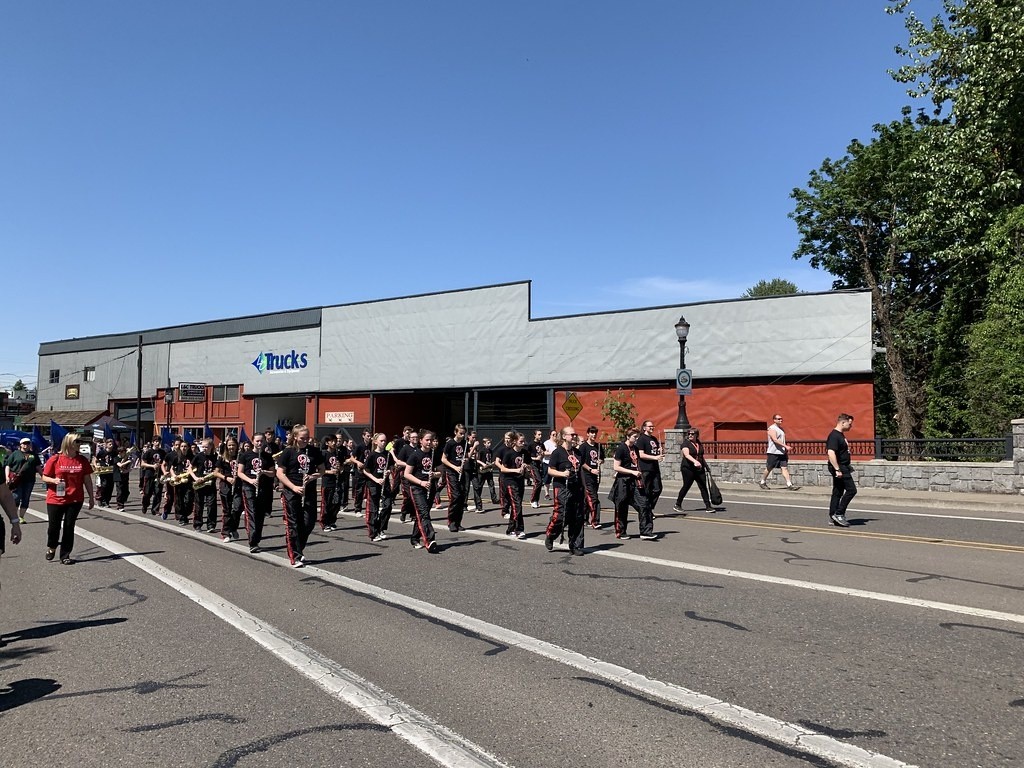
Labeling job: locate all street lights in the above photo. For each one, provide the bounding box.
[674,315,692,430]
[16,396,22,430]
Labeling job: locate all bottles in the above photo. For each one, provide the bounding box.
[57,479,65,496]
[96,475,101,485]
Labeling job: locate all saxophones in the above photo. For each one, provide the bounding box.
[170,471,189,487]
[193,472,215,490]
[272,450,283,458]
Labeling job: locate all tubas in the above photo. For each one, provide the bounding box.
[119,444,139,470]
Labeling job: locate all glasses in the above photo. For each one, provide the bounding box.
[687,432,695,435]
[564,433,578,437]
[775,418,782,421]
[74,439,84,445]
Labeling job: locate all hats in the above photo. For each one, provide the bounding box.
[19,437,31,444]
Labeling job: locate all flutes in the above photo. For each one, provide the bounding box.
[228,429,675,508]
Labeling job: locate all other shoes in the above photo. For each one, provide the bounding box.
[503,513,511,520]
[546,539,553,550]
[788,485,800,490]
[674,505,684,513]
[339,505,483,522]
[292,556,305,567]
[428,540,437,554]
[508,531,526,540]
[98,501,262,553]
[640,534,658,539]
[706,508,715,513]
[372,531,386,542]
[573,548,584,556]
[758,481,770,491]
[45,547,56,561]
[324,524,337,532]
[59,558,71,564]
[620,534,630,540]
[584,521,601,530]
[414,542,423,550]
[450,523,465,531]
[530,501,540,508]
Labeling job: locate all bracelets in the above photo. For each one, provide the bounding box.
[835,468,840,472]
[10,517,20,524]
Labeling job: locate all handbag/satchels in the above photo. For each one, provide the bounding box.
[706,471,723,505]
[8,471,20,483]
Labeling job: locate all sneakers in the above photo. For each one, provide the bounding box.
[828,510,851,527]
[19,516,27,524]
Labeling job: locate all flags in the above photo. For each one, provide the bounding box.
[50,418,69,451]
[104,421,113,439]
[239,427,250,443]
[184,429,195,444]
[275,423,287,443]
[32,422,50,451]
[130,431,136,446]
[161,427,178,448]
[205,421,213,439]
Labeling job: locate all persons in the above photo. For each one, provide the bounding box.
[758,414,801,491]
[92,420,665,569]
[42,431,94,565]
[826,413,857,527]
[0,461,22,648]
[42,450,51,490]
[5,438,43,524]
[672,428,716,513]
